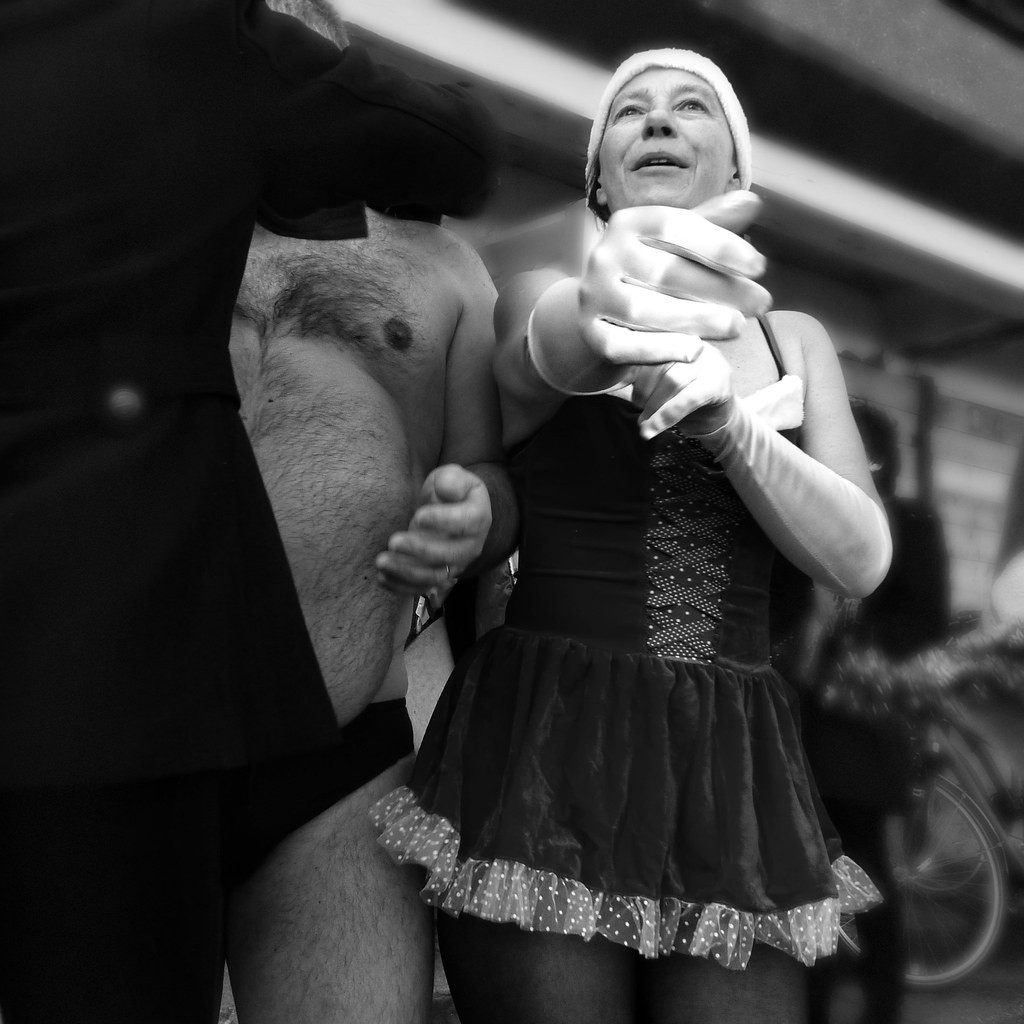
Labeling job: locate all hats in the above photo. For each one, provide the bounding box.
[582,42,753,225]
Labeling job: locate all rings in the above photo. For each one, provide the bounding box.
[443,563,451,581]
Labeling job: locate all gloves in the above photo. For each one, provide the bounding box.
[526,190,774,394]
[617,343,893,600]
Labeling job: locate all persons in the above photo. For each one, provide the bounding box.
[0,1,499,1024]
[222,0,514,1024]
[370,46,884,1024]
[772,399,951,1024]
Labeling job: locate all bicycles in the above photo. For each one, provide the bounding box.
[823,618,1024,990]
